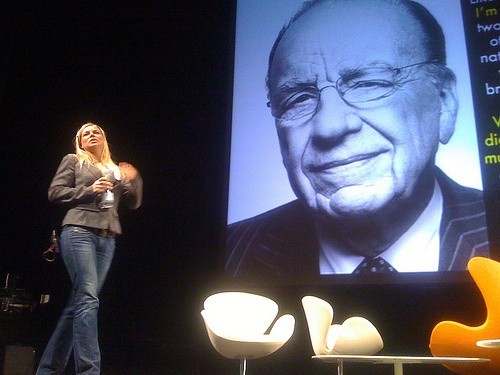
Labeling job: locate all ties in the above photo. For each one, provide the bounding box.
[350,257,399,274]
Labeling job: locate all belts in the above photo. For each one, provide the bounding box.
[73,225,118,239]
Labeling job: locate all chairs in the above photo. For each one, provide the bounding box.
[201,292,295,375]
[301,295,384,375]
[429,257,500,375]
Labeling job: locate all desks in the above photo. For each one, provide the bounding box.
[312,354,491,375]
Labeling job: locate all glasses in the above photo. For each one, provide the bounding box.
[266,58,440,120]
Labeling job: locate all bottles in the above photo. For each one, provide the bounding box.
[102,171,114,208]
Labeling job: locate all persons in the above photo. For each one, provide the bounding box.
[225,0,489,276]
[36,122,143,375]
